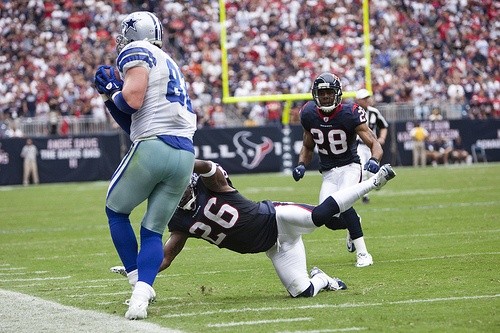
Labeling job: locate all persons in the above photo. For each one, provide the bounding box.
[93,9,198,320]
[20,138,40,185]
[428,137,473,166]
[352,88,389,201]
[288,73,383,269]
[0,0,500,136]
[111,158,397,300]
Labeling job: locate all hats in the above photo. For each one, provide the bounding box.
[356,88,371,101]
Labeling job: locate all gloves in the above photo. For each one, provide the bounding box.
[93,65,112,92]
[363,159,380,174]
[293,164,305,181]
[108,265,127,278]
[96,66,124,99]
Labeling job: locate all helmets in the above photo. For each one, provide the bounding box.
[120,11,163,47]
[312,73,343,113]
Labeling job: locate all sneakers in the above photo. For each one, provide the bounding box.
[125,280,156,319]
[375,164,394,191]
[310,266,348,291]
[355,252,373,267]
[346,216,362,252]
